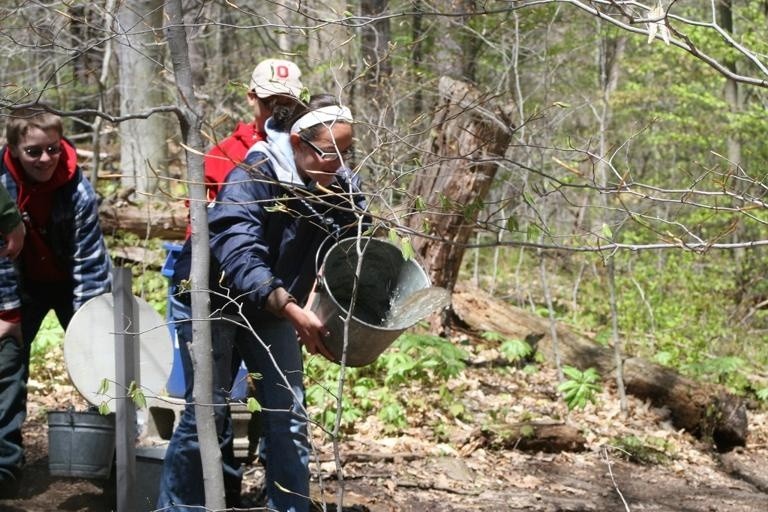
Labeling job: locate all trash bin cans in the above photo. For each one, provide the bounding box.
[161,242,251,398]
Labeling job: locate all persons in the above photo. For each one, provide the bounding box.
[0,105,117,501]
[185,59,310,247]
[1,181,28,264]
[155,90,375,511]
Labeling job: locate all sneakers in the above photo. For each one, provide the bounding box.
[1,473,18,499]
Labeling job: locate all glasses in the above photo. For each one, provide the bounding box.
[26,143,60,157]
[300,135,353,162]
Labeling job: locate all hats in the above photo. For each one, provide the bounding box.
[250,58,304,99]
